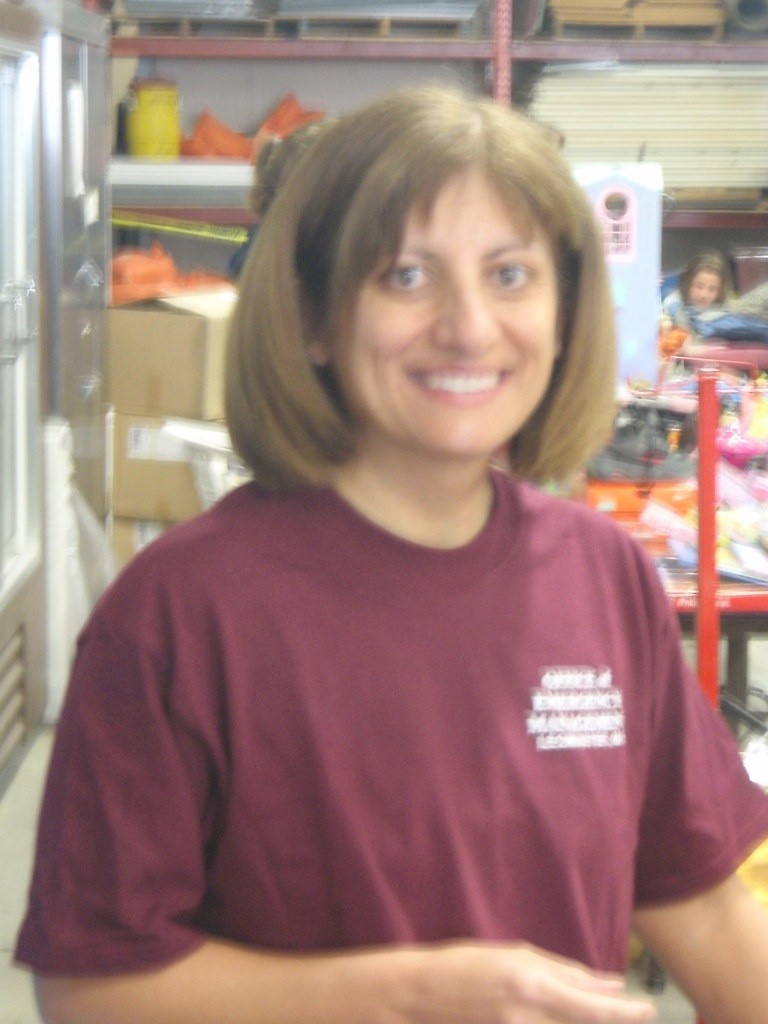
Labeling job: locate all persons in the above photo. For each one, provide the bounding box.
[10,87,768,1024]
[228,118,334,269]
[660,254,726,322]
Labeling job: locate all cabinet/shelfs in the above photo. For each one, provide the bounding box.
[111,40,768,227]
[0,0,114,796]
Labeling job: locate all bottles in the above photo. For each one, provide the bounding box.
[665,413,682,451]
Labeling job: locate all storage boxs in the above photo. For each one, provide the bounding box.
[110,287,251,573]
